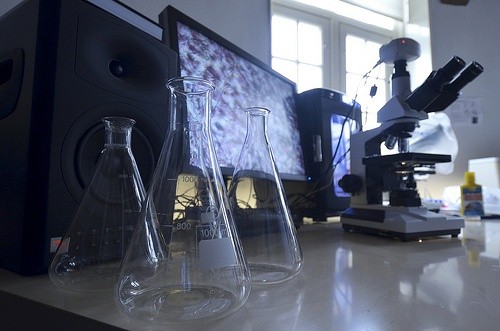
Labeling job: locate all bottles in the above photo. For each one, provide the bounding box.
[111,73,252,324]
[204,106,302,285]
[48,117,173,301]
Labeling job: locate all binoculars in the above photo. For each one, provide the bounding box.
[340,37,484,243]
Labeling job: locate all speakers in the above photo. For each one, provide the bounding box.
[0,0,179,273]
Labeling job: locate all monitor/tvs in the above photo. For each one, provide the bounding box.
[158,4,314,193]
